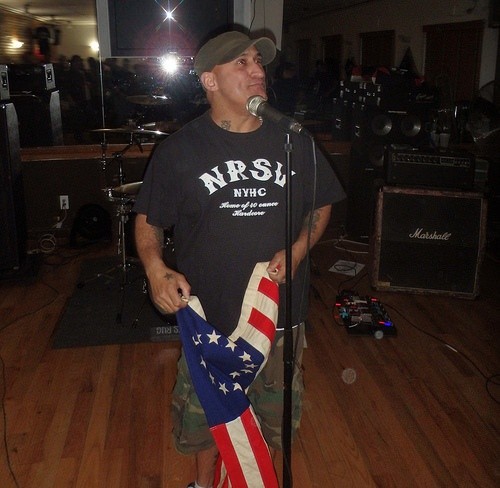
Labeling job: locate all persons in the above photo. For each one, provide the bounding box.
[131,31,350,488]
[8,53,209,145]
[265,54,299,113]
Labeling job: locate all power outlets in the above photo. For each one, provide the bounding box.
[59,195,69,209]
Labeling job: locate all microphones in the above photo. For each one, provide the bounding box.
[245,94,313,138]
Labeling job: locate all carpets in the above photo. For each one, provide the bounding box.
[50,254,181,348]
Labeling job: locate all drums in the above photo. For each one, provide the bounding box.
[102,189,141,205]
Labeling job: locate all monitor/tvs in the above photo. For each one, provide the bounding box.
[96,0,251,58]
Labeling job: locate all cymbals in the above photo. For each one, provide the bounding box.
[143,121,184,131]
[127,94,173,106]
[91,127,170,137]
[116,181,145,194]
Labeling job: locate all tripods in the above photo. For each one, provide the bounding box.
[77,132,145,324]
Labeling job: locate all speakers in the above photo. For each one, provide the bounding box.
[13,91,66,147]
[345,108,433,241]
[0,102,29,280]
[369,181,489,302]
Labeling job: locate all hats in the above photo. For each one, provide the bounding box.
[194,32,277,77]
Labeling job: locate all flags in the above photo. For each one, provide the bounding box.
[175,261,281,488]
